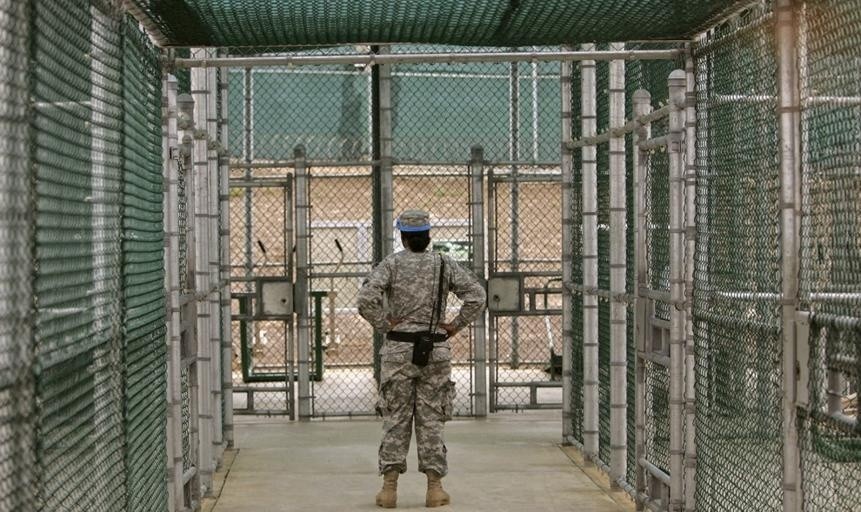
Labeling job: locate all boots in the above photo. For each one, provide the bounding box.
[376,470,399,508]
[426,470,450,507]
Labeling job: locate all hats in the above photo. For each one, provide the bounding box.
[396,210,431,232]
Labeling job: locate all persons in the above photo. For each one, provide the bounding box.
[356,209,488,509]
